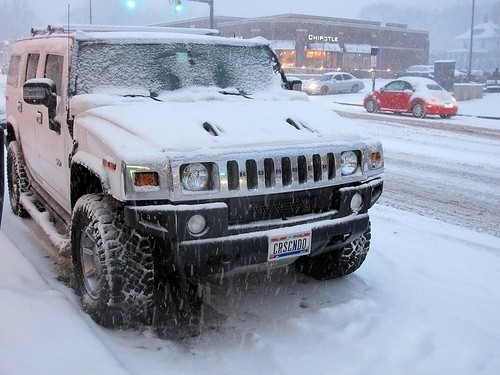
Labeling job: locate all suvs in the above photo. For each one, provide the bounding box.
[0,25,384,329]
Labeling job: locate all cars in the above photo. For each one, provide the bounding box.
[363,76,458,119]
[301,73,365,95]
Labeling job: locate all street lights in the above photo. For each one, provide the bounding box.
[175,0,214,34]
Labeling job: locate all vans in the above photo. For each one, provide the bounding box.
[395,65,433,78]
